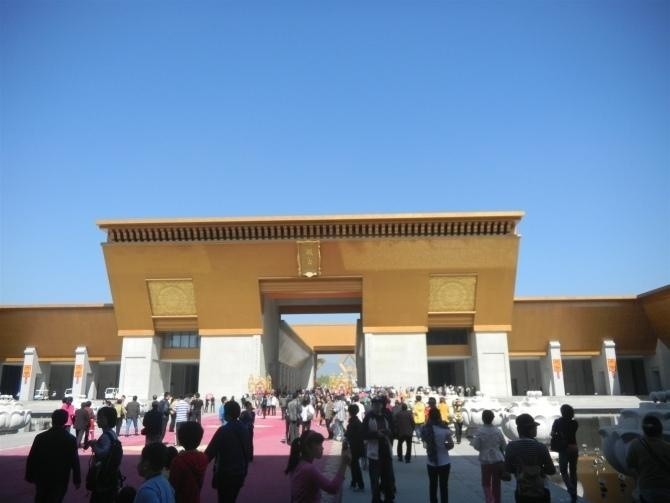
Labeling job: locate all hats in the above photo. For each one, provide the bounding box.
[516,414,540,426]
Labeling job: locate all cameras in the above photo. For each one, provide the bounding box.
[342,440,351,450]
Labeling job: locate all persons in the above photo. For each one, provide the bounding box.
[624,414,670,503]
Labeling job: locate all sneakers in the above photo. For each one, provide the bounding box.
[349,484,365,492]
[398,456,411,463]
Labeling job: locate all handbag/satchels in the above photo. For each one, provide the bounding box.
[551,438,560,451]
[104,440,122,471]
[86,471,116,492]
[497,462,510,481]
[342,438,352,464]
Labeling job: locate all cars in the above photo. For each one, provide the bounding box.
[104,387,117,400]
[115,387,119,399]
[64,387,72,400]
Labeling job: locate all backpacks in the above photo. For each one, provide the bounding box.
[512,440,545,497]
